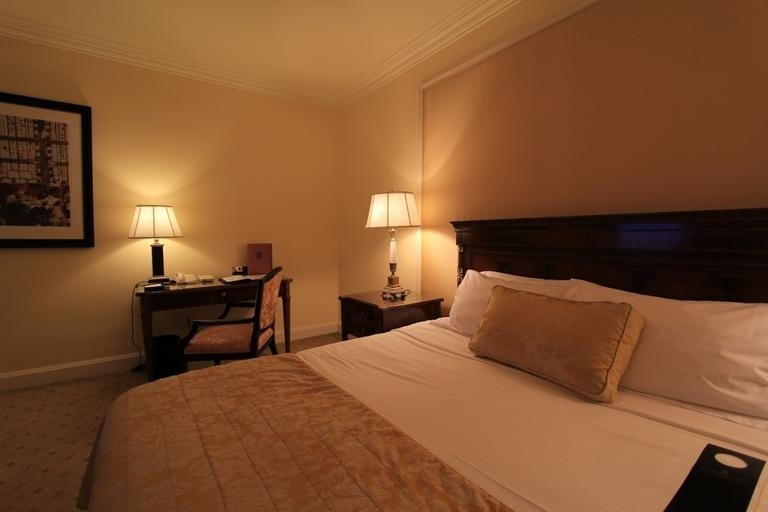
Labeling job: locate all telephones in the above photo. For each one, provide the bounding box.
[175,273,196,283]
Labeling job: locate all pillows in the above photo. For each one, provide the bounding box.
[448,269,571,337]
[562,283,767,418]
[469,285,644,405]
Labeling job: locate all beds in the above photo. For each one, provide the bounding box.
[77,208,768,512]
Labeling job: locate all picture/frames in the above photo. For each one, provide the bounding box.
[0,92,95,248]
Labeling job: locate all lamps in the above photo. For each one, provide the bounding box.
[365,191,422,302]
[128,205,183,277]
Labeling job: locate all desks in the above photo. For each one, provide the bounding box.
[134,273,293,382]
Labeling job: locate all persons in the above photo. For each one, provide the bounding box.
[2,195,28,225]
[23,200,53,225]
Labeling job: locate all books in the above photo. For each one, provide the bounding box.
[218,271,267,284]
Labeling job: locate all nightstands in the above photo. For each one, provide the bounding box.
[338,289,443,342]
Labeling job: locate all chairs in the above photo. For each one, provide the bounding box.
[176,266,282,374]
